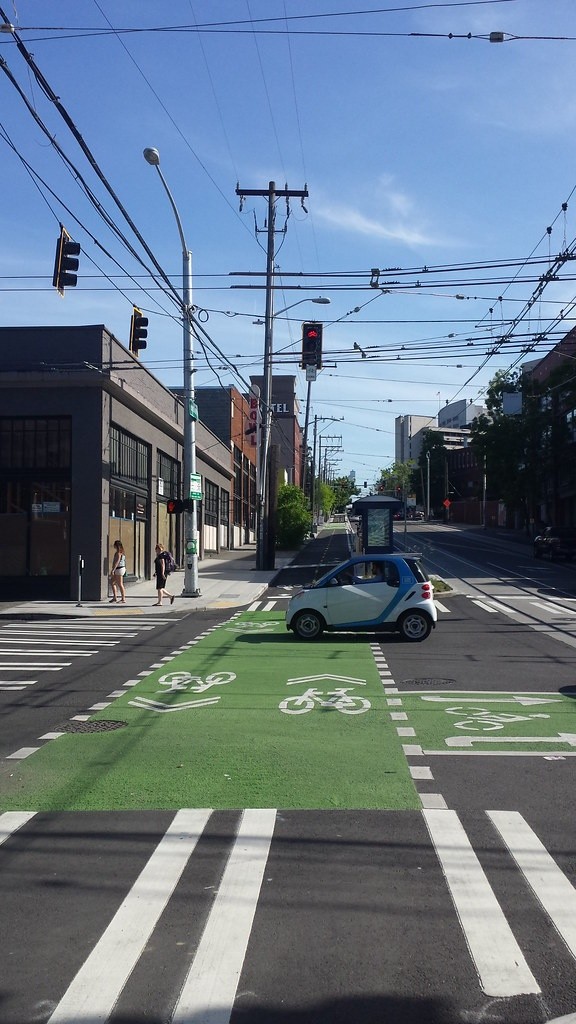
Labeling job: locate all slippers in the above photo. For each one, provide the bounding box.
[171,596,175,604]
[153,604,162,606]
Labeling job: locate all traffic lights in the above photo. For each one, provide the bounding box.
[379,485,385,492]
[300,322,322,370]
[396,486,401,492]
[55,228,81,300]
[129,307,149,357]
[166,499,185,516]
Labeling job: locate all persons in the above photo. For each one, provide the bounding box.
[109,540,127,604]
[345,562,384,583]
[151,543,175,606]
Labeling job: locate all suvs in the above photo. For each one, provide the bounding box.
[533,525,576,563]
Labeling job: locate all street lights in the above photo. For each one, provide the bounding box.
[144,146,203,598]
[255,297,332,571]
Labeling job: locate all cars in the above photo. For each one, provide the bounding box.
[393,507,444,520]
[285,551,438,644]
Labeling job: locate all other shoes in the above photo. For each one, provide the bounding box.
[116,600,126,603]
[108,599,117,603]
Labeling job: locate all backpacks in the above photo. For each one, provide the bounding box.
[158,550,175,573]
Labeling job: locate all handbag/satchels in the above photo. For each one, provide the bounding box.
[123,571,127,577]
